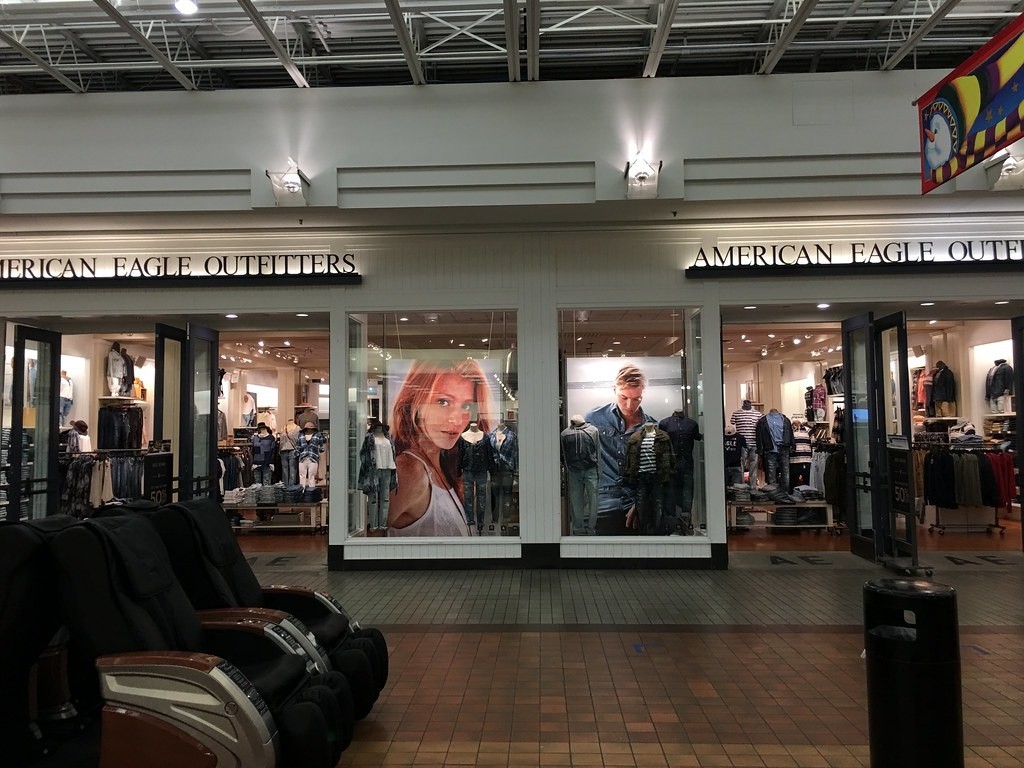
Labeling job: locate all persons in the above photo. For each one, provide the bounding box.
[489,422,518,524]
[106,347,135,396]
[730,400,764,490]
[560,415,602,533]
[804,383,827,421]
[383,358,491,537]
[757,408,795,490]
[59,370,73,427]
[933,362,957,417]
[359,418,399,537]
[298,407,321,431]
[625,423,676,535]
[250,422,276,486]
[295,422,325,488]
[584,363,660,535]
[243,392,256,423]
[923,367,939,418]
[278,419,303,485]
[456,422,498,531]
[985,359,1015,414]
[658,409,704,535]
[724,424,749,487]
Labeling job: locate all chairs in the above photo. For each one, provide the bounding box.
[0,496,388,768]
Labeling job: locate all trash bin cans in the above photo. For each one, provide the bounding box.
[862,577,966,768]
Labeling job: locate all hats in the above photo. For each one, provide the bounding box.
[70,420,88,436]
[255,422,267,430]
[299,422,318,433]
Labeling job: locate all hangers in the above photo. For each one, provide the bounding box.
[100,402,143,412]
[59,449,147,464]
[810,447,851,463]
[912,435,1011,459]
[218,448,252,462]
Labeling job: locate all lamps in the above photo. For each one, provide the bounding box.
[249,344,300,364]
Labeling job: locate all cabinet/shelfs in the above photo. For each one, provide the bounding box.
[728,500,834,536]
[983,412,1021,507]
[223,498,329,537]
